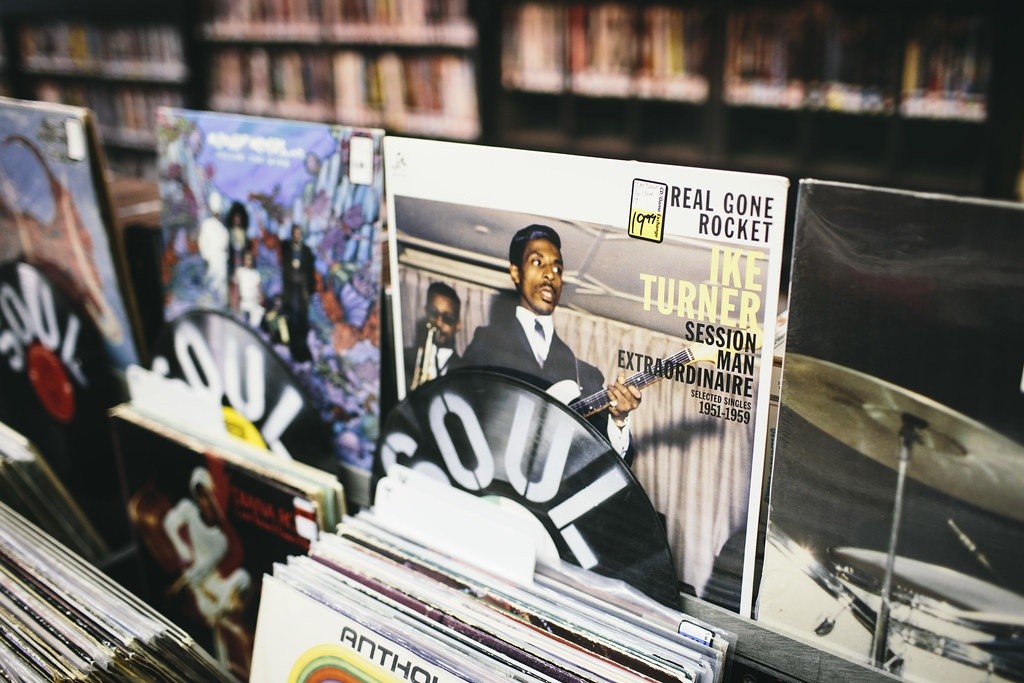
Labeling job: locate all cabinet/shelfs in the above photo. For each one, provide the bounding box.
[24,0,1024,184]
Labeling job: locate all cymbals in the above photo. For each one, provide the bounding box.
[780,352,1024,523]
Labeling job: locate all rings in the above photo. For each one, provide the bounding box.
[610,400,617,406]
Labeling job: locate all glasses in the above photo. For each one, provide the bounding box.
[426,304,456,326]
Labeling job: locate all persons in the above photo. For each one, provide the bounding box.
[460,224,642,467]
[404,282,461,394]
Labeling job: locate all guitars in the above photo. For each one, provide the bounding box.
[544,325,764,419]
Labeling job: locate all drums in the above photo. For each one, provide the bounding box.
[828,545,1024,648]
[886,621,1024,682]
[756,520,903,672]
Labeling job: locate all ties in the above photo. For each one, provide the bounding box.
[534,319,546,341]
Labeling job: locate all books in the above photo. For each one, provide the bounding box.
[202,0,469,119]
[498,0,709,106]
[721,0,995,122]
[18,20,185,131]
